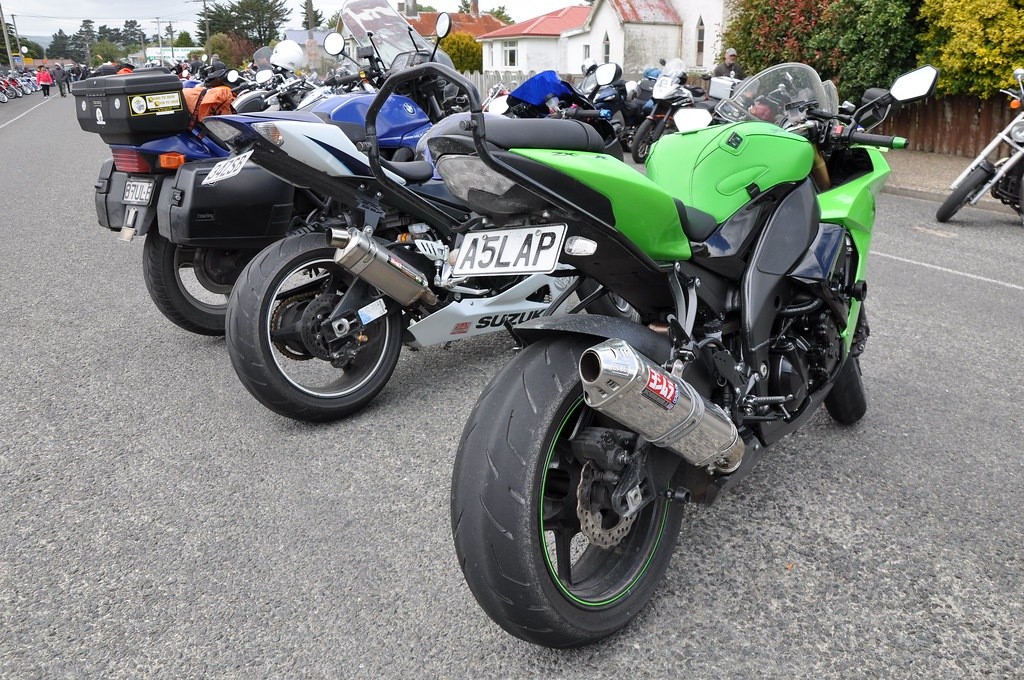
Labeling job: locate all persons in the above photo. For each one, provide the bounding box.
[65,60,129,83]
[52,63,69,96]
[169,51,210,82]
[36,65,53,98]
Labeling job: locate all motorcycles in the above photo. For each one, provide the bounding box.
[0,0,1024,650]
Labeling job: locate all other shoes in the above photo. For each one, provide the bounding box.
[45,96,49,98]
[69,91,71,93]
[62,94,66,97]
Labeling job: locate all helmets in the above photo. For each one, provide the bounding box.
[269,40,304,71]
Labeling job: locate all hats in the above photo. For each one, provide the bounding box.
[212,54,220,59]
[726,48,737,55]
